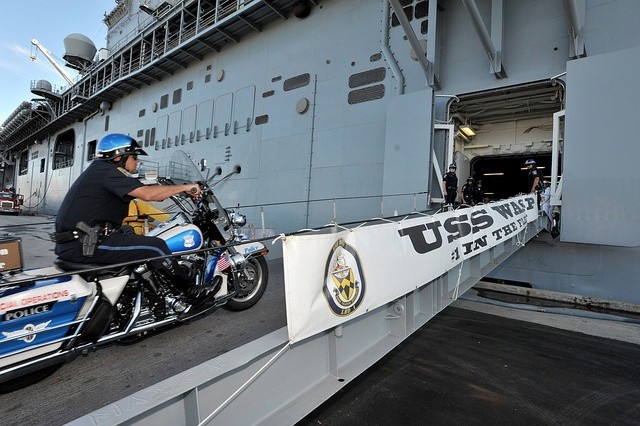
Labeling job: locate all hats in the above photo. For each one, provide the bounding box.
[450,162,454,167]
[525,157,535,164]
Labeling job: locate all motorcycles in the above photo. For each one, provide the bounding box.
[0,149,268,382]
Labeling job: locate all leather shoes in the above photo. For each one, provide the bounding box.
[187,275,223,309]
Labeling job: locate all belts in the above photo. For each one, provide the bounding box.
[50,230,86,242]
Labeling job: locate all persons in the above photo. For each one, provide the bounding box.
[523,158,542,192]
[542,180,550,196]
[473,179,486,202]
[49,132,224,308]
[461,176,475,206]
[442,163,458,209]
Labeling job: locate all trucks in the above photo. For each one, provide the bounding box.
[0,191,24,214]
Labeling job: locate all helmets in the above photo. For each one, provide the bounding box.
[94,133,148,161]
